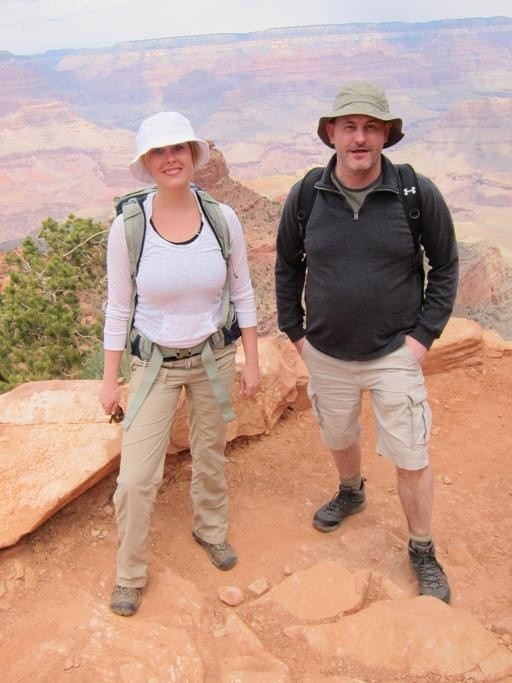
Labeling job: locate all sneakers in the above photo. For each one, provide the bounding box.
[195,534,237,570]
[408,543,452,604]
[111,587,139,616]
[315,481,368,533]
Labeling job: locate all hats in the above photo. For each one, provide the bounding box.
[129,111,208,183]
[316,81,404,149]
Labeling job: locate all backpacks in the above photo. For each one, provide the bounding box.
[113,184,239,362]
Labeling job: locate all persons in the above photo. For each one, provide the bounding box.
[96,108,262,616]
[274,79,458,605]
